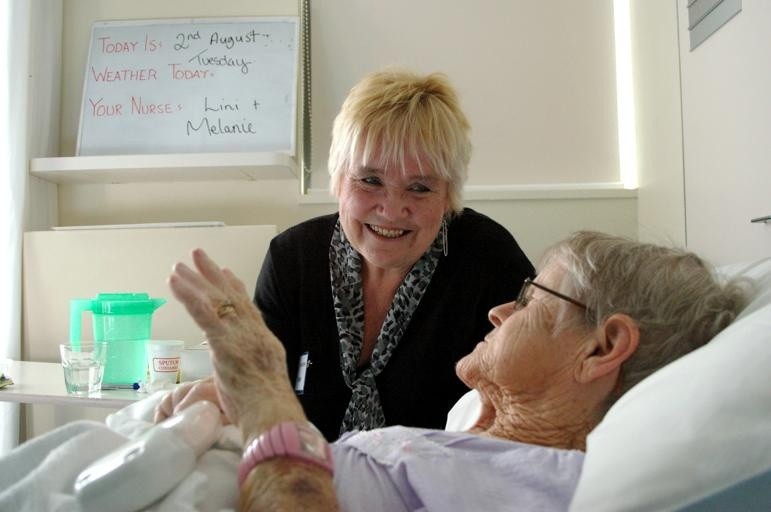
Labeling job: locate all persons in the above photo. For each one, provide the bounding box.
[253,65,537,444]
[153,230,763,512]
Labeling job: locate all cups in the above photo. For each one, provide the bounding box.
[67,290,168,389]
[59,341,108,397]
[145,338,183,388]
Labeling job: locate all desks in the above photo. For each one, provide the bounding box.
[0,358,150,409]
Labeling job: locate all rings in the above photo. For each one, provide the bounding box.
[217,304,235,317]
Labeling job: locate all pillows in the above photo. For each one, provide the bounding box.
[444,264,771,511]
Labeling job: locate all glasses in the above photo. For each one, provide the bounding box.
[515,278,590,313]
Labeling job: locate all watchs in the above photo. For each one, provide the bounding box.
[238,418,336,489]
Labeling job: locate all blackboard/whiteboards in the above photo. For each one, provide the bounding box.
[75,16,301,157]
